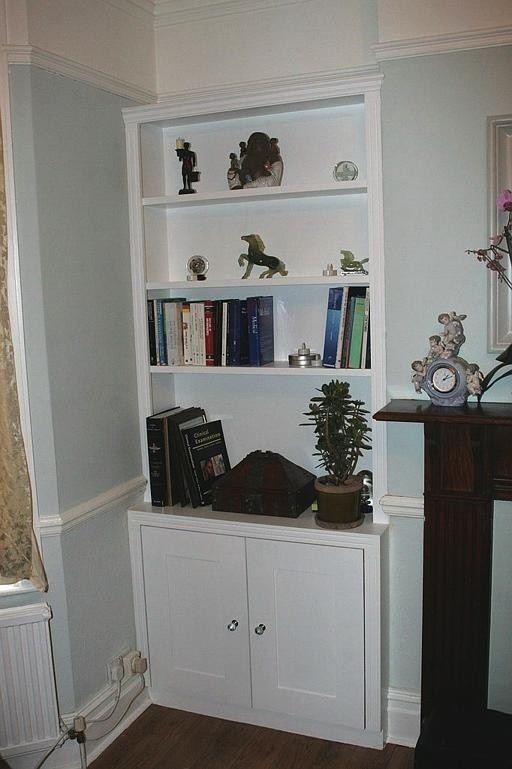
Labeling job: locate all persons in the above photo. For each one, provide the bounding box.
[270,137,280,155]
[438,313,461,351]
[229,152,239,170]
[411,360,428,394]
[178,142,196,190]
[425,335,443,358]
[466,363,484,396]
[239,141,247,157]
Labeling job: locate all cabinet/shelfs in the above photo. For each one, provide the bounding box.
[128,500,390,751]
[118,74,383,519]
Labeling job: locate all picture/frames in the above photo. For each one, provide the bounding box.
[487,113,512,354]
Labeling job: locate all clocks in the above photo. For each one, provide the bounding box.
[411,312,482,407]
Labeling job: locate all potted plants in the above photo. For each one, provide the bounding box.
[298,379,372,529]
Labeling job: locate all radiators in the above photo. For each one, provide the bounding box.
[0,603,65,759]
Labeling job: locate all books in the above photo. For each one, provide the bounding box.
[145,404,233,508]
[322,286,370,369]
[147,294,274,367]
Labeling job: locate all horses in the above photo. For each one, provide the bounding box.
[238,235,288,279]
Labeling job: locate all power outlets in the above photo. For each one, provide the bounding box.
[105,647,139,684]
